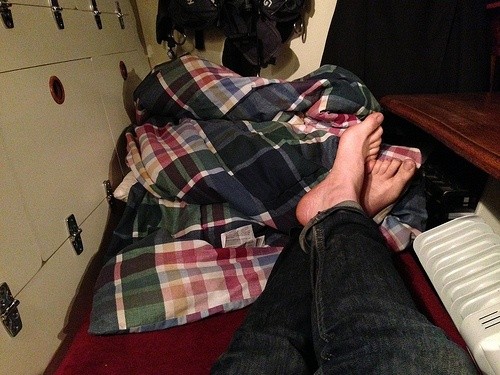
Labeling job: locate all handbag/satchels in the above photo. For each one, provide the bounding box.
[156,0,310,76]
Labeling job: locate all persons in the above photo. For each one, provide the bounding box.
[212,113,480,375]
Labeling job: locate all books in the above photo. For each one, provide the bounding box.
[406,145,494,217]
